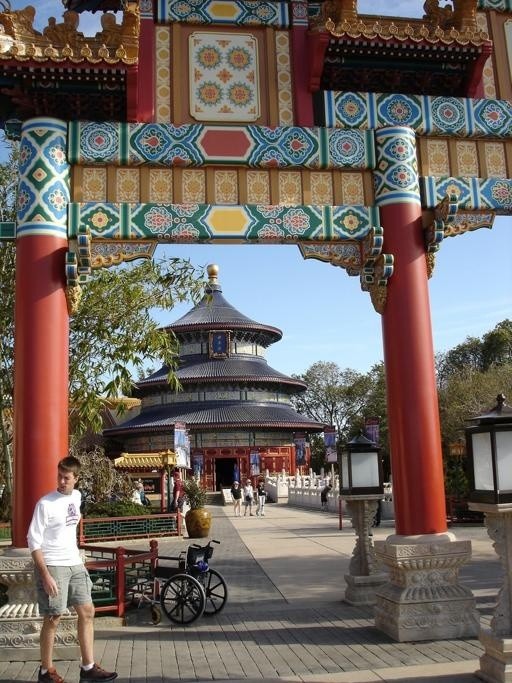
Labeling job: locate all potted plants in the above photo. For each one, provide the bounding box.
[178,477,211,537]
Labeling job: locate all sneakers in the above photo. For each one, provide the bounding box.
[37,665,66,683]
[79,663,118,683]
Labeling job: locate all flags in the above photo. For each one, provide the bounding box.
[364,415,379,447]
[193,452,204,475]
[294,438,307,465]
[174,420,192,470]
[249,450,261,476]
[323,425,337,463]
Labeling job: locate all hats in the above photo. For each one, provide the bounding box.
[232,478,265,485]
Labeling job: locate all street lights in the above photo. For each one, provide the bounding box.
[464,393,512,683]
[339,430,388,607]
[159,449,176,513]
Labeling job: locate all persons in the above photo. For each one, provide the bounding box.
[26,453,118,682]
[131,480,143,505]
[231,480,242,518]
[256,478,268,516]
[170,472,185,514]
[243,478,255,516]
[138,482,146,506]
[320,483,333,512]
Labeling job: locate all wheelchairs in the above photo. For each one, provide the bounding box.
[138,540,227,624]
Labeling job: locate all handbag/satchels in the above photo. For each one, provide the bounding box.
[245,495,251,501]
